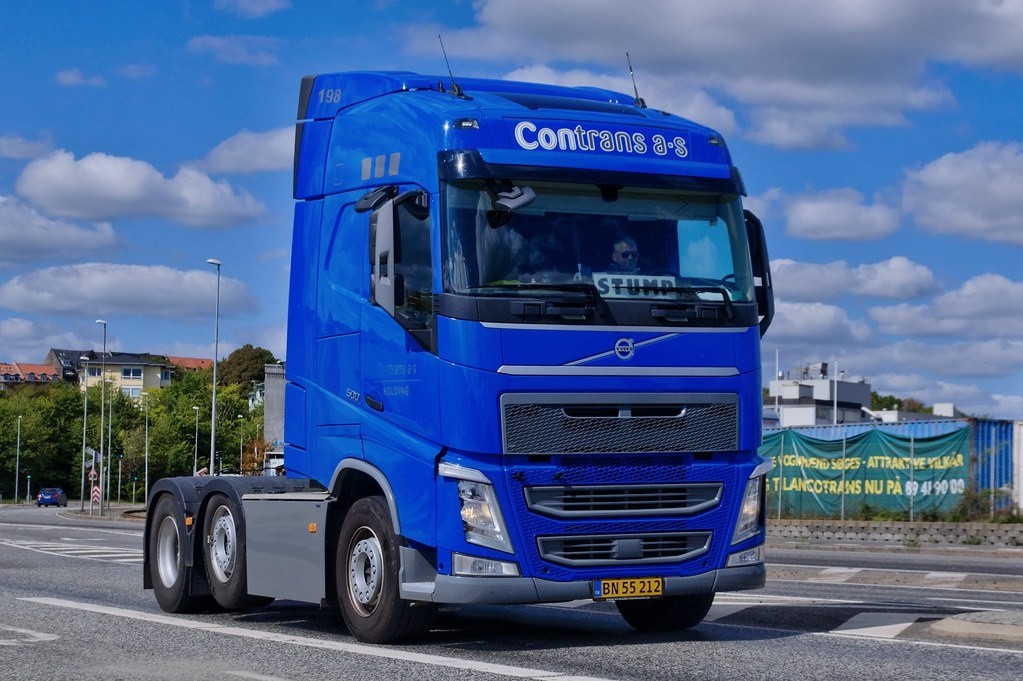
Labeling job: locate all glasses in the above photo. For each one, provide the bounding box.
[614,250,639,259]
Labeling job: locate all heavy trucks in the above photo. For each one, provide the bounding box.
[142,64,776,646]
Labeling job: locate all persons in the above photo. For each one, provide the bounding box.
[609,236,641,274]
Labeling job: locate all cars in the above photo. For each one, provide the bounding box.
[217,467,240,476]
[36,487,68,507]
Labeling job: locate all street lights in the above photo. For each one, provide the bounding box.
[192,406,199,476]
[96,318,107,516]
[141,391,149,506]
[104,379,113,512]
[206,257,222,476]
[237,414,244,475]
[80,355,89,512]
